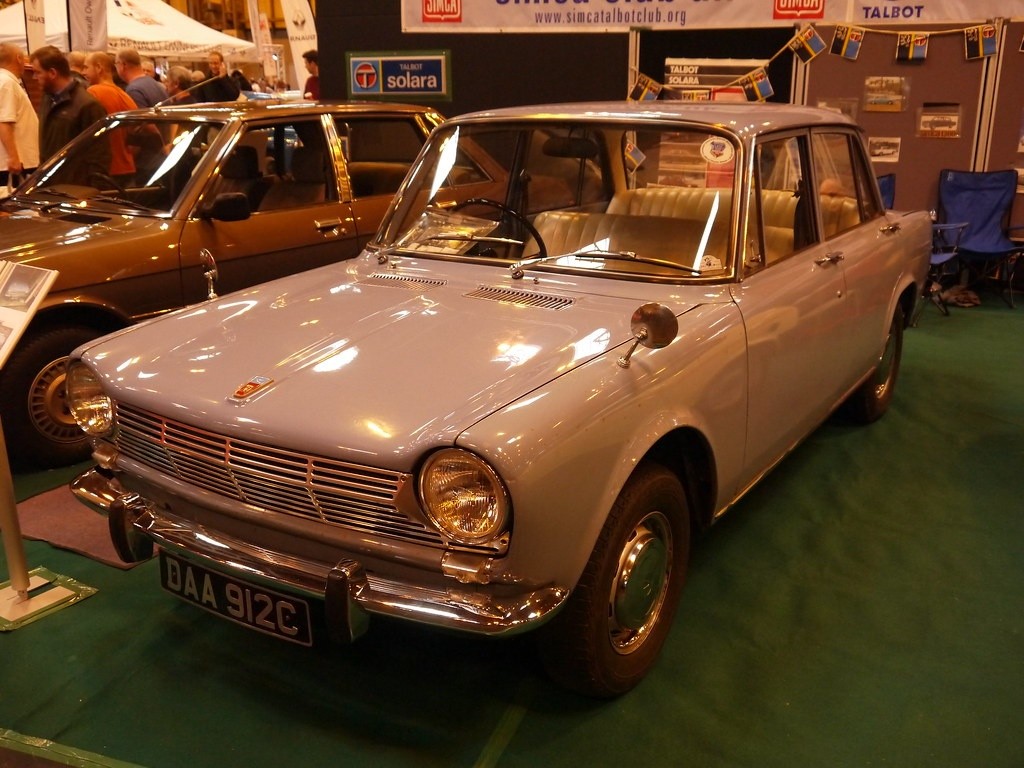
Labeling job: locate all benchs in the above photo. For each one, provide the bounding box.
[518,209,795,277]
[603,187,861,269]
[344,160,469,200]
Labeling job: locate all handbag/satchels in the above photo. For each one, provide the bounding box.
[0,173,24,199]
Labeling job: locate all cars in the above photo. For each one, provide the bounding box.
[0,102,454,469]
[64,100,935,703]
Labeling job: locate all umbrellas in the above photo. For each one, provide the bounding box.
[0,0,259,65]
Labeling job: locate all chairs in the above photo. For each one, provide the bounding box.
[255,145,336,211]
[199,144,265,212]
[875,169,1024,327]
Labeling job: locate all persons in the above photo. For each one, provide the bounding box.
[165,65,197,104]
[67,50,166,188]
[191,53,289,103]
[212,144,297,214]
[0,41,40,189]
[303,49,321,101]
[29,45,110,189]
[107,50,167,109]
